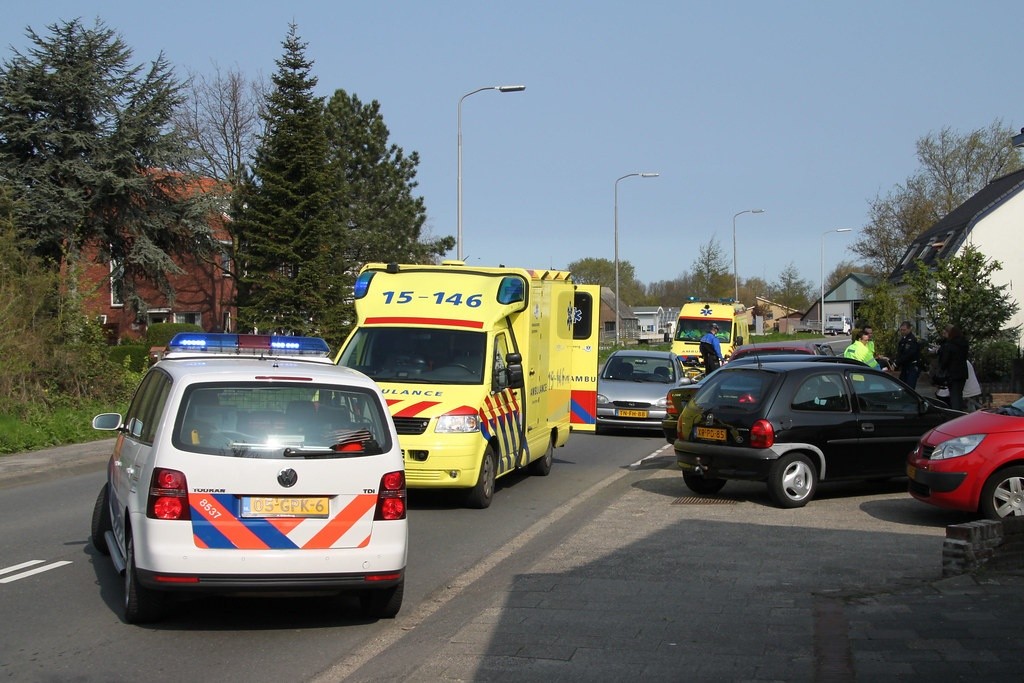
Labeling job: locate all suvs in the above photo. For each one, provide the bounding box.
[673,361,970,508]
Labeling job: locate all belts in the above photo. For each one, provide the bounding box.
[911,363,919,366]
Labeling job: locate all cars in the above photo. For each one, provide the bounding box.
[906,396,1024,519]
[595,349,691,433]
[661,354,949,445]
[728,342,827,363]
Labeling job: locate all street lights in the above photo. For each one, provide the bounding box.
[821,228,852,336]
[734,208,765,300]
[457,85,526,263]
[615,173,660,344]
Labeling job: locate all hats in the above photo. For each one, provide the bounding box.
[711,323,720,331]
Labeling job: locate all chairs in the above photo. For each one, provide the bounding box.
[819,382,846,409]
[615,362,634,380]
[285,401,351,443]
[653,367,670,380]
[446,335,481,374]
[384,334,427,370]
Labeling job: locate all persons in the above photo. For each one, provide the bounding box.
[891,321,919,392]
[843,324,889,393]
[699,322,726,376]
[938,323,982,413]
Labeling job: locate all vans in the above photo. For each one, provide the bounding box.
[91,333,407,625]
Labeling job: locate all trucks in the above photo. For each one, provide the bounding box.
[333,261,601,508]
[791,313,852,336]
[671,297,750,379]
